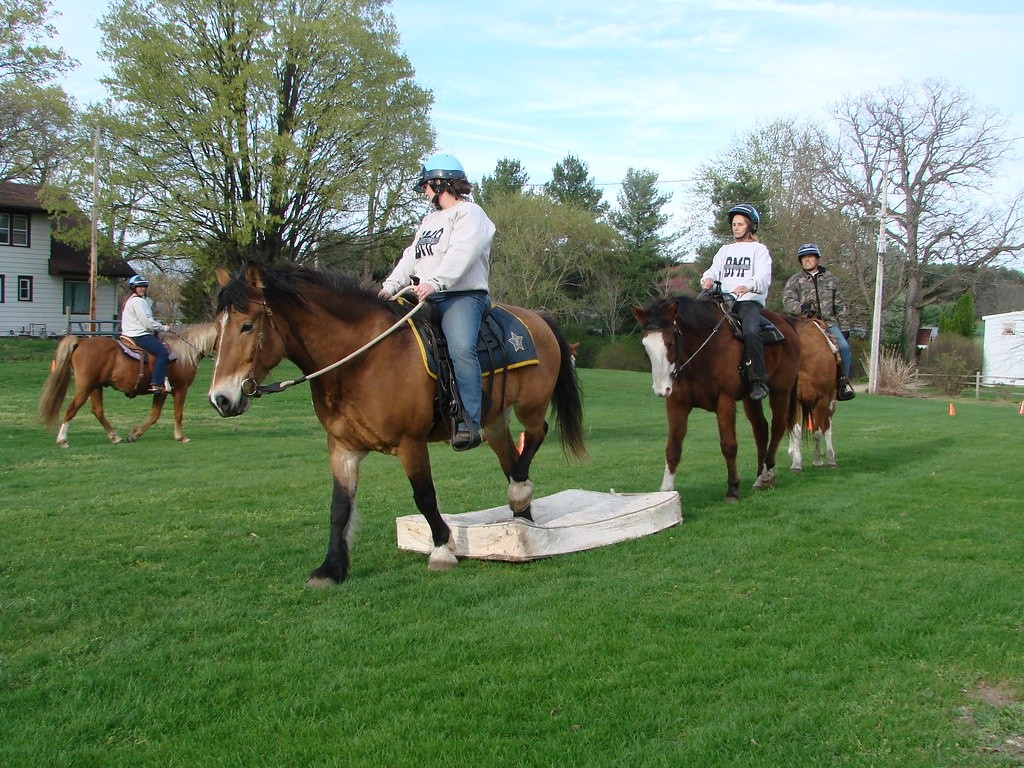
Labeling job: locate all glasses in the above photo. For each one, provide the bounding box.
[422,183,428,190]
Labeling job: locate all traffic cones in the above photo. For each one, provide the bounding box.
[1019,400,1024,414]
[949,403,956,416]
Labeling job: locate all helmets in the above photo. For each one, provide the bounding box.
[728,204,759,223]
[129,275,149,288]
[412,154,465,193]
[797,244,821,262]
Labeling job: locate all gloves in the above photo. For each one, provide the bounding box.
[842,331,850,340]
[801,303,811,311]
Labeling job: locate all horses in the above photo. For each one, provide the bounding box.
[35,322,218,449]
[628,296,839,504]
[208,259,590,587]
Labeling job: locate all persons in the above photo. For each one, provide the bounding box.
[701,204,773,400]
[378,153,497,445]
[782,244,854,402]
[121,274,171,392]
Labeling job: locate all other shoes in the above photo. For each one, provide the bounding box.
[841,388,855,397]
[454,431,487,448]
[151,384,174,389]
[751,383,770,400]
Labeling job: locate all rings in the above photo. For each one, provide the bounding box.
[421,290,426,296]
